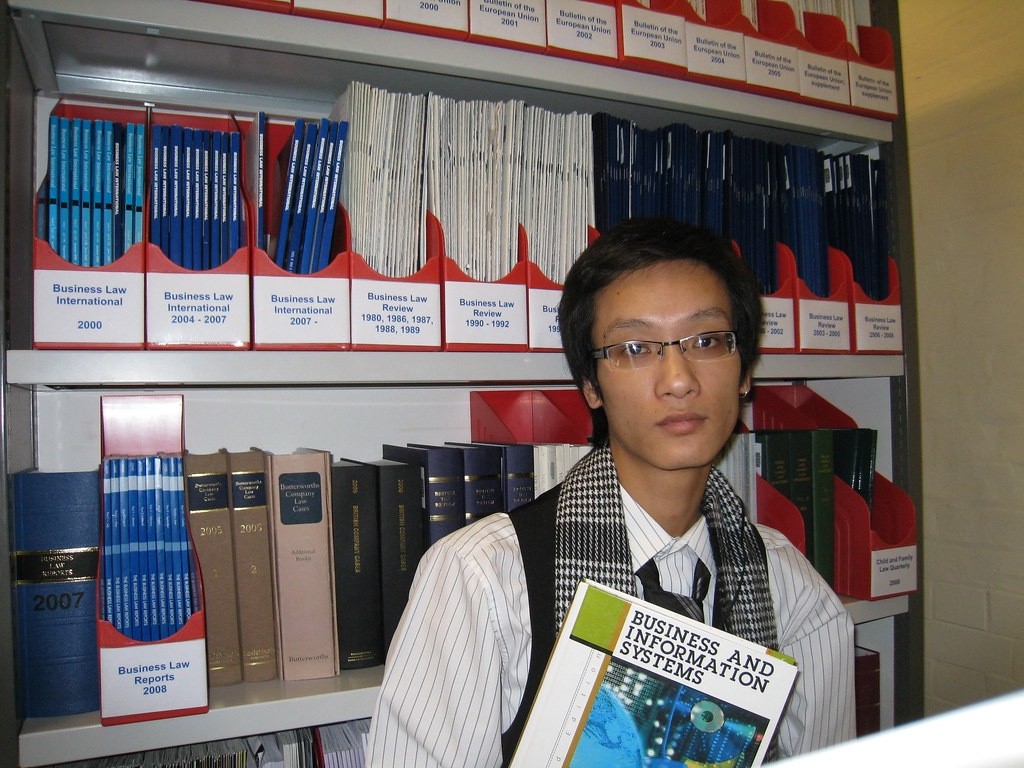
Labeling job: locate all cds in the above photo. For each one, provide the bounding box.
[664,685,757,763]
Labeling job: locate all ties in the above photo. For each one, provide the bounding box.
[635,556,712,624]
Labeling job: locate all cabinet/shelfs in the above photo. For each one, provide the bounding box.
[0,0,920,768]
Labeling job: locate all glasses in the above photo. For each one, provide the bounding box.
[590,329,737,370]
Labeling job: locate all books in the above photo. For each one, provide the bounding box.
[0,0,891,768]
[510,578,799,768]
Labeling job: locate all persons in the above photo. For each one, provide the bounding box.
[368,214,856,768]
